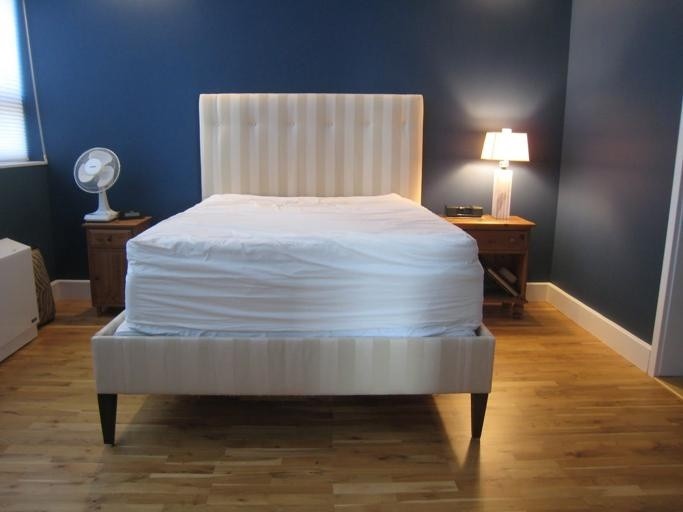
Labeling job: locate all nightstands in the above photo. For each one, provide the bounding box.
[440,215,536,319]
[82,216,153,317]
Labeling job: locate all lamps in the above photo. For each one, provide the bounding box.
[480,127,530,221]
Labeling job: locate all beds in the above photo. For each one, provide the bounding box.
[90,93,496,447]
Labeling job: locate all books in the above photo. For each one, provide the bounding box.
[486,269,520,298]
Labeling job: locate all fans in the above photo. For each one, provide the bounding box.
[73,147,121,223]
